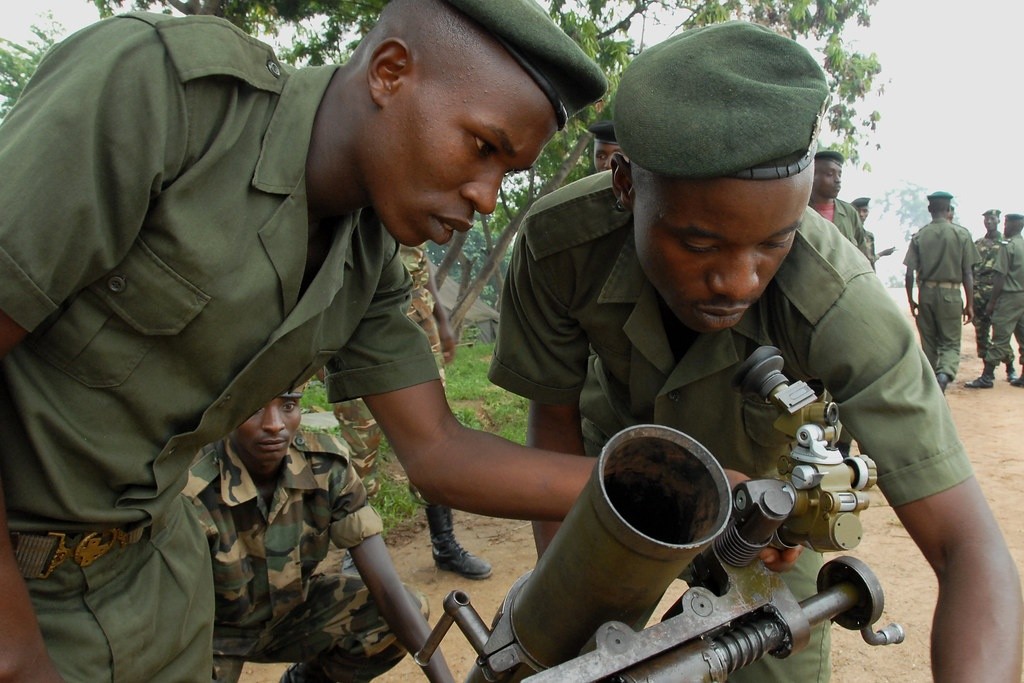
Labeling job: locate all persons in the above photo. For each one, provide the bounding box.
[587,121,629,172]
[972,209,1019,382]
[850,197,897,264]
[317,241,494,579]
[964,214,1024,388]
[903,191,983,395]
[180,380,457,683]
[807,152,876,273]
[0,0,804,683]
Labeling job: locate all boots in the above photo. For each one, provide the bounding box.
[965,363,994,388]
[425,503,493,580]
[1006,362,1015,381]
[937,372,950,392]
[1011,365,1024,387]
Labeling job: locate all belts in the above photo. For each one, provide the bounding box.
[925,282,960,289]
[11,522,144,579]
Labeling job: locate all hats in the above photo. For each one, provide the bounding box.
[815,151,844,168]
[928,191,952,204]
[982,209,1001,216]
[852,197,870,208]
[589,120,618,145]
[612,20,831,180]
[445,0,608,133]
[1004,214,1024,220]
